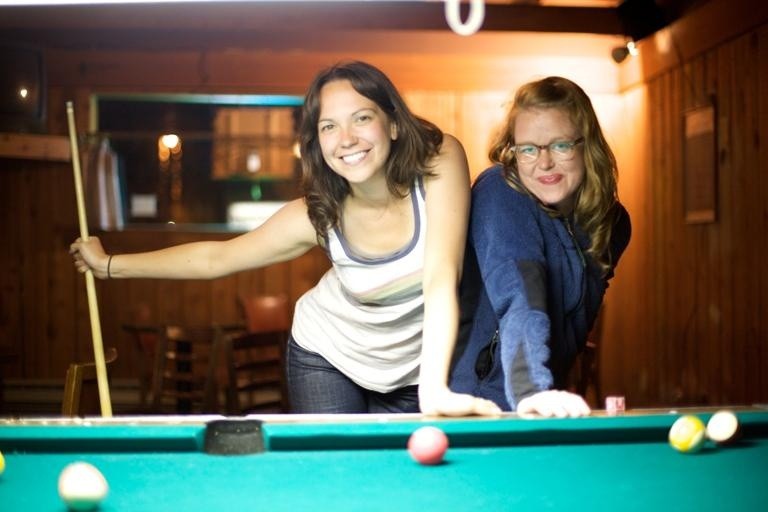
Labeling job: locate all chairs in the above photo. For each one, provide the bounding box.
[148,291,291,415]
[61,346,120,412]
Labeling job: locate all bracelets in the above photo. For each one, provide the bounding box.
[108,254,113,281]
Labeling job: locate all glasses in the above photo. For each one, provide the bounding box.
[511,137,583,164]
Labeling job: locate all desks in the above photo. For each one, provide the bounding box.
[0,402,767,512]
[125,322,246,413]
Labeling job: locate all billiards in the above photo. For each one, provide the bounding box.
[708,412,741,443]
[669,416,705,453]
[59,461,107,510]
[407,427,448,464]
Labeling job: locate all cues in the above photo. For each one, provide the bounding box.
[67,101,114,416]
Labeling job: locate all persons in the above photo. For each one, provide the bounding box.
[68,60,502,417]
[448,77,631,419]
